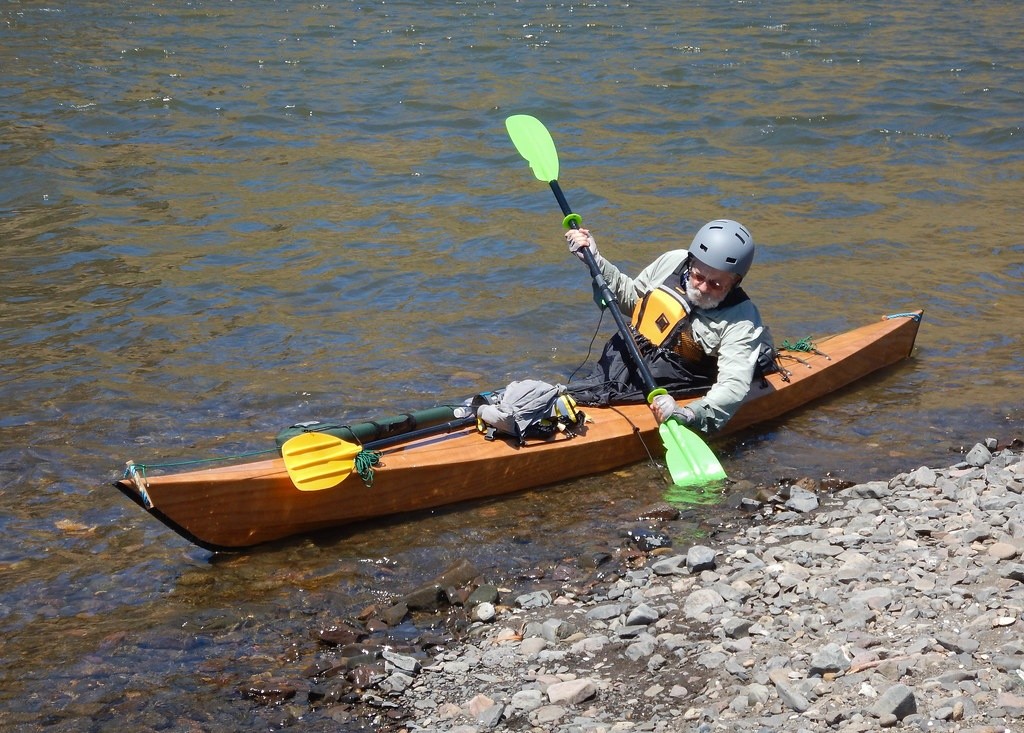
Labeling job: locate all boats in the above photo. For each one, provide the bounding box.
[110,307,928,555]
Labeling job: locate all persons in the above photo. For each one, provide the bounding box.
[564,219,764,437]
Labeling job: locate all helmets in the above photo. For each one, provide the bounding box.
[688,219,755,289]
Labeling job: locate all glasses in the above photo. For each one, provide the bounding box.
[689,270,723,292]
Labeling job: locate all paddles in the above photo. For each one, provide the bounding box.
[504,112,728,490]
[280,416,478,493]
